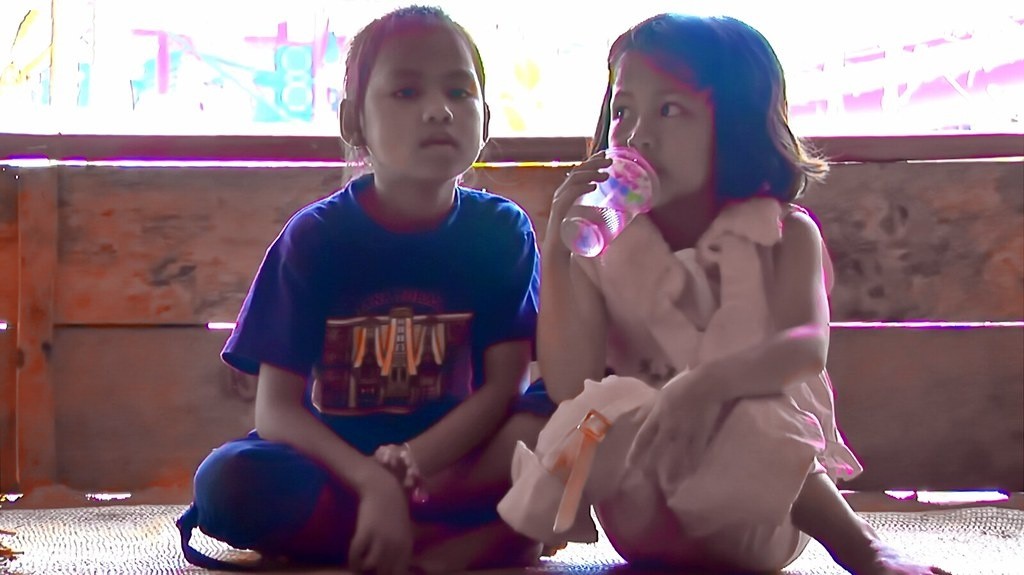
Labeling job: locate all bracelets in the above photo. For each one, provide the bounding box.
[376,441,419,489]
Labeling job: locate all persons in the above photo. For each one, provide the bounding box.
[413,13,954,575]
[194,6,557,574]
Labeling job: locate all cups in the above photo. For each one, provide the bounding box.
[559,147,662,259]
